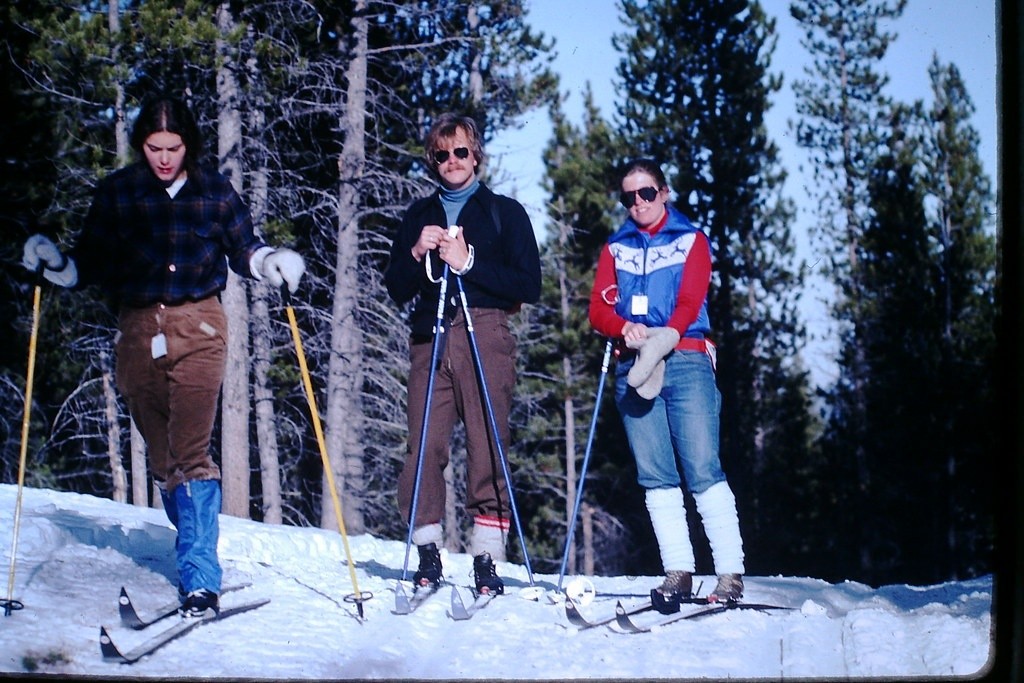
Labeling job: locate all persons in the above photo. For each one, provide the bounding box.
[587,158,746,612]
[383,112,543,597]
[22,91,306,619]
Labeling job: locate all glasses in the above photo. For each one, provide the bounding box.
[619,187,661,209]
[431,147,473,165]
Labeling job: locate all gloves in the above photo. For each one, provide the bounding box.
[251,246,306,294]
[624,326,680,388]
[22,234,63,271]
[634,359,665,400]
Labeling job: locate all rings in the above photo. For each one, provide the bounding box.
[430,237,433,242]
[440,249,445,255]
[636,335,641,338]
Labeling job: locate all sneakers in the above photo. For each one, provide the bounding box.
[413,542,447,590]
[178,588,220,617]
[655,570,692,600]
[469,551,504,595]
[710,574,744,603]
[177,582,187,602]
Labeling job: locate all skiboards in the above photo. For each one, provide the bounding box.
[394,581,499,620]
[564,605,738,634]
[99,581,270,664]
